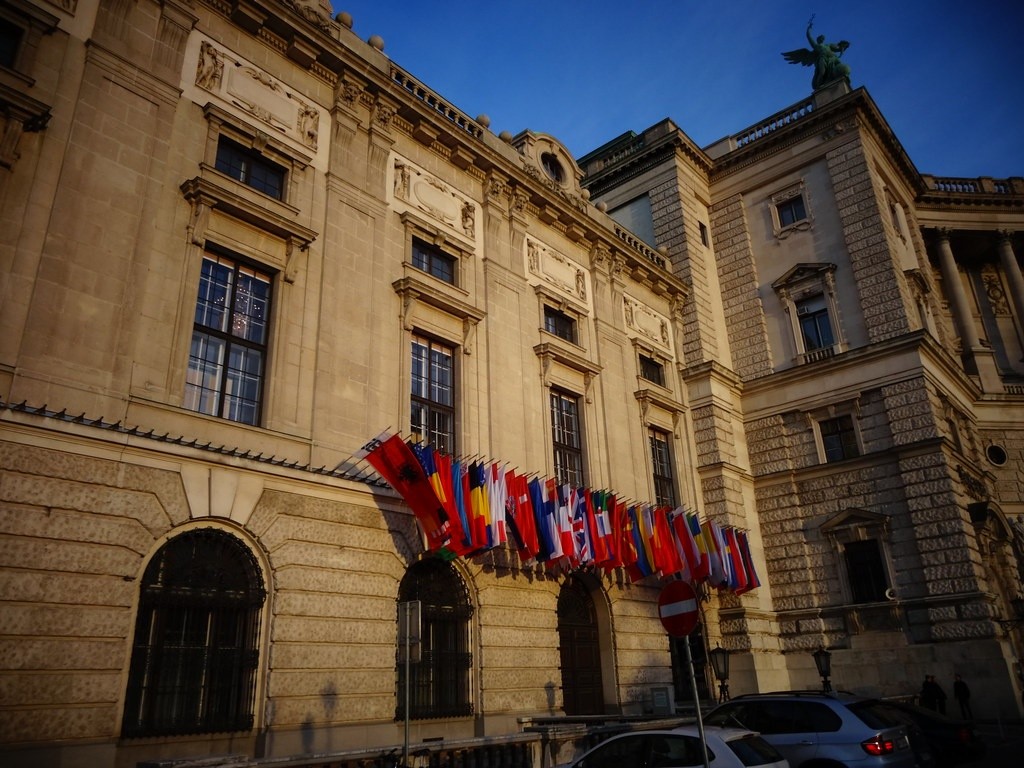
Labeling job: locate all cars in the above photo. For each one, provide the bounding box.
[555,725,789,768]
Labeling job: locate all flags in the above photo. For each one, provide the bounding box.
[351,427,761,596]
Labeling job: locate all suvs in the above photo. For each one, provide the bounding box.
[694,688,940,768]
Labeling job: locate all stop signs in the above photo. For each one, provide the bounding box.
[658,581,700,638]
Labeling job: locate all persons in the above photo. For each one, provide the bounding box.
[662,323,668,342]
[393,162,404,198]
[922,675,946,716]
[780,24,851,89]
[954,673,973,720]
[624,301,631,325]
[196,41,214,88]
[528,240,538,274]
[463,206,475,238]
[577,269,584,299]
[300,109,317,148]
